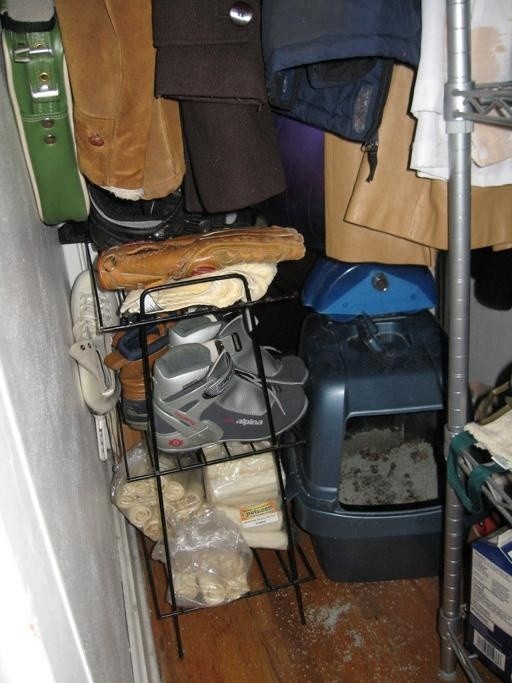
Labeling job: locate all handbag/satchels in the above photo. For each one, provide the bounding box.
[3,21,91,225]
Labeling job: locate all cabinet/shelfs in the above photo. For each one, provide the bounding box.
[438,1,512,683]
[82,230,317,659]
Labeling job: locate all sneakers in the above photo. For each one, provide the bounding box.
[168,313,309,387]
[149,343,310,454]
[104,309,183,430]
[70,270,120,417]
